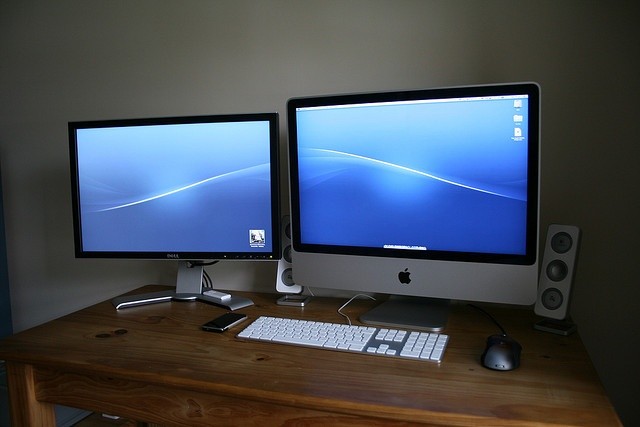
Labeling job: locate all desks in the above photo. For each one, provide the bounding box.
[1,284,622,427]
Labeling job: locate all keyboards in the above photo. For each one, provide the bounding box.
[235,315,449,364]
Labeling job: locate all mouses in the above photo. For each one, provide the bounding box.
[480,334,524,372]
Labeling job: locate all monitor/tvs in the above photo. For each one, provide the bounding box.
[285,80,540,334]
[67,112,283,309]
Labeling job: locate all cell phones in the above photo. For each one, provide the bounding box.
[199,309,249,333]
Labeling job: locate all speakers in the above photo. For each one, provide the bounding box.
[275,209,311,307]
[534,225,582,337]
